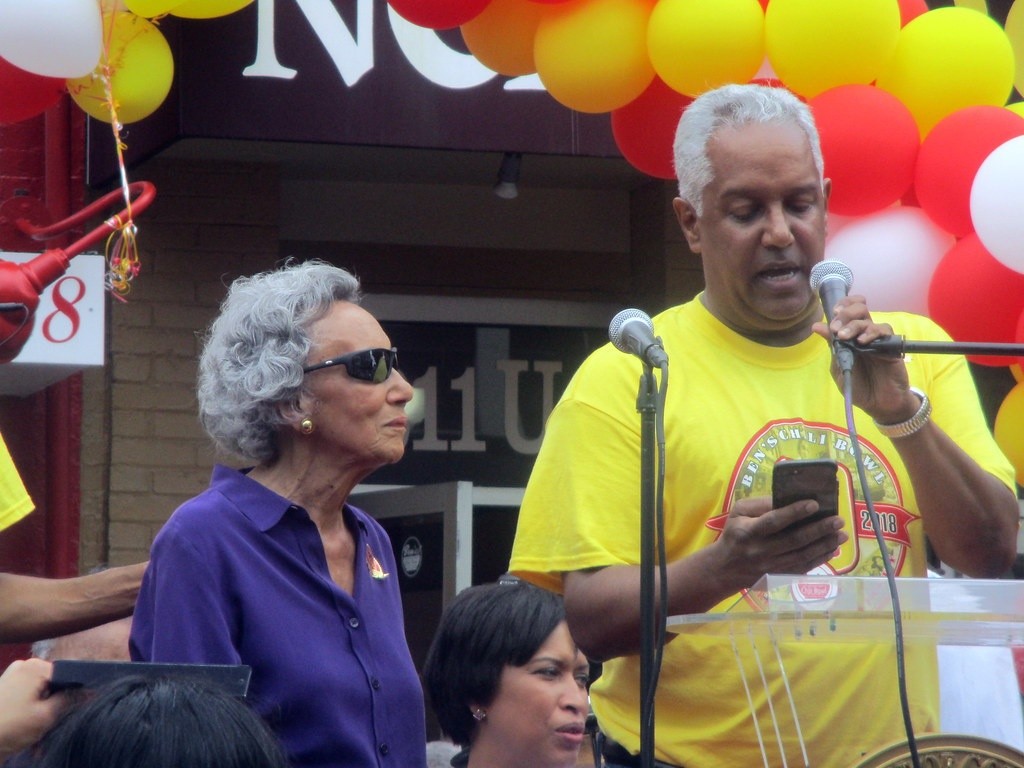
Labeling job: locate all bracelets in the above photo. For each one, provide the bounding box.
[872,386,932,438]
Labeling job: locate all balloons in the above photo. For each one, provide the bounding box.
[0,0,1024,493]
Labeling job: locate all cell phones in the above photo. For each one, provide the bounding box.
[773,457,839,556]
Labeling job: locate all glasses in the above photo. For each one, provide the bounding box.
[304,348,397,384]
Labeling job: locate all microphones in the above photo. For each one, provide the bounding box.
[809,259,856,374]
[608,308,668,369]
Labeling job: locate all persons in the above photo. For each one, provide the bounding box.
[0,659,287,768]
[510,84,1020,768]
[423,583,588,768]
[131,265,427,768]
[0,428,150,644]
